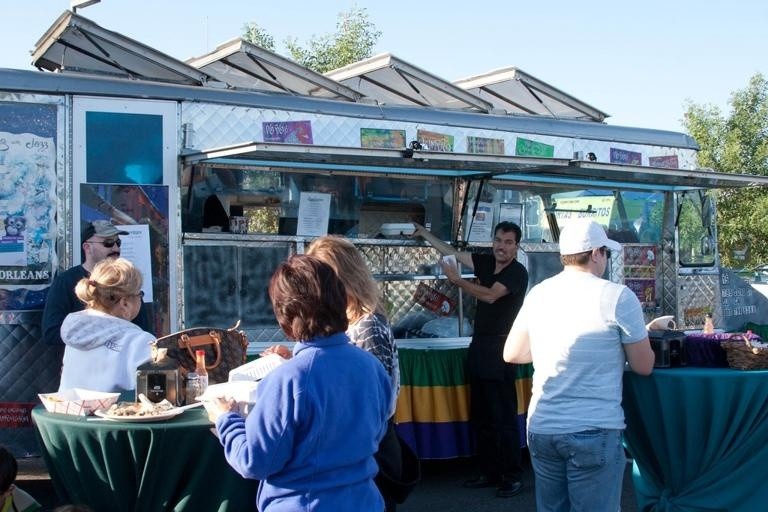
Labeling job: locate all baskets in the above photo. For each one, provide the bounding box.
[721,335,768,369]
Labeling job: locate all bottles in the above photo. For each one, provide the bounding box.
[195,349,208,404]
[184,372,201,405]
[745,330,754,339]
[703,312,714,333]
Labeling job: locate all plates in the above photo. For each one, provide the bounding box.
[93,406,185,423]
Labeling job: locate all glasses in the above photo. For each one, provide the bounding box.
[606,249,611,259]
[103,239,120,247]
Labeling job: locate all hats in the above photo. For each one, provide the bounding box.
[81,220,129,242]
[559,220,621,255]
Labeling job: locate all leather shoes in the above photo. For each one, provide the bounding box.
[464,477,523,497]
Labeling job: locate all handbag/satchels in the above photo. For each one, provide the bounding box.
[157,320,248,385]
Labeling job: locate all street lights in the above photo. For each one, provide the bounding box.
[70,0,102,14]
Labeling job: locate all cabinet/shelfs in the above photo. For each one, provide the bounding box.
[182,177,480,335]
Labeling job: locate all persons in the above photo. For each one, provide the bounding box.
[196,253,393,511]
[40,219,149,367]
[59,258,157,407]
[401,219,531,497]
[259,233,419,510]
[501,219,658,512]
[0,445,45,512]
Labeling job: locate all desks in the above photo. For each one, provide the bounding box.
[32,334,768,510]
